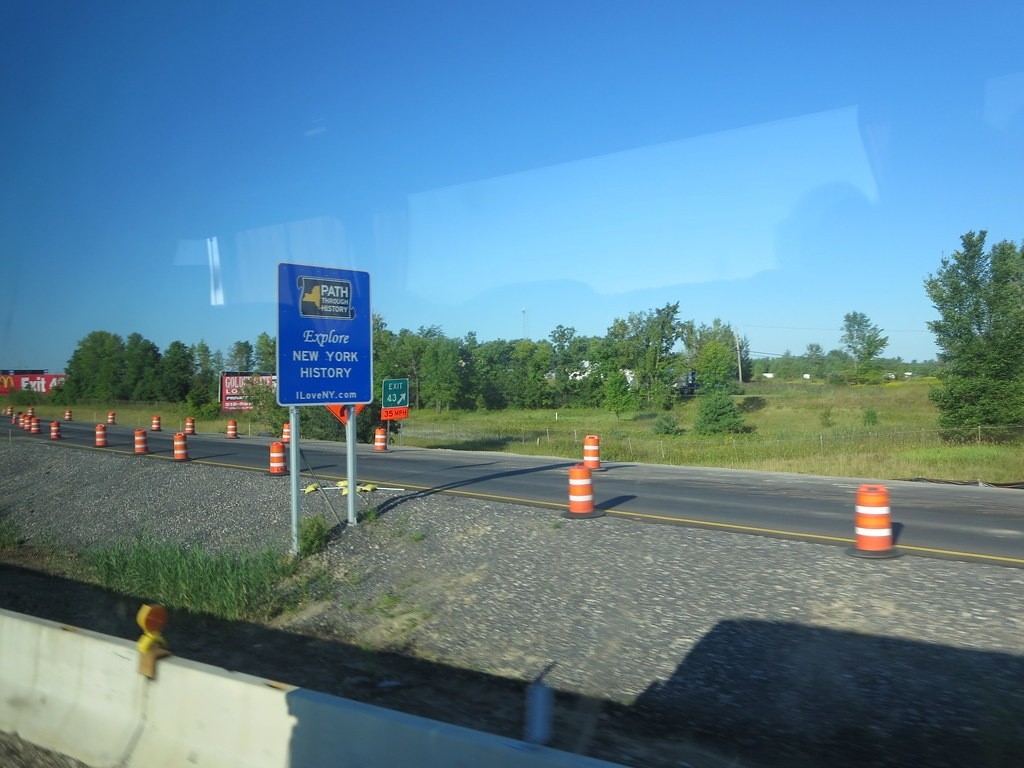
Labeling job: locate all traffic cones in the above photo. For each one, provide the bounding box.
[12,414,16,424]
